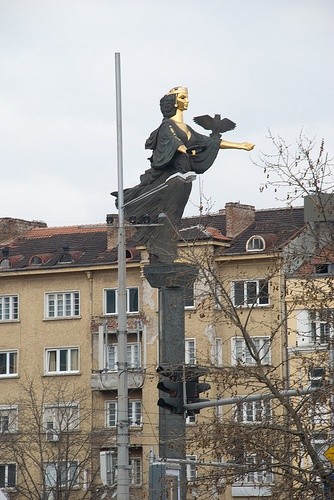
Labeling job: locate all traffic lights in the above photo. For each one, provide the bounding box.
[155,365,212,415]
